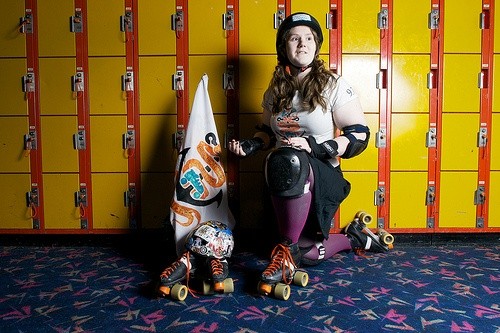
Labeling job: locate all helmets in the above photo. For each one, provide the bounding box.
[184,219,234,259]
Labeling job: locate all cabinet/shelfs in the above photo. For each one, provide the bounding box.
[1,0,500,238]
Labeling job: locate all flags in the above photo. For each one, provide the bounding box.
[170,73,235,256]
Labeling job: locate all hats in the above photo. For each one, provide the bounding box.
[276,11,323,54]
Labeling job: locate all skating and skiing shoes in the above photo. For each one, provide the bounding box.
[255,236,309,301]
[202,256,234,294]
[343,211,395,255]
[157,250,198,300]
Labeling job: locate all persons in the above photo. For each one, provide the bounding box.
[228,12,394,300]
